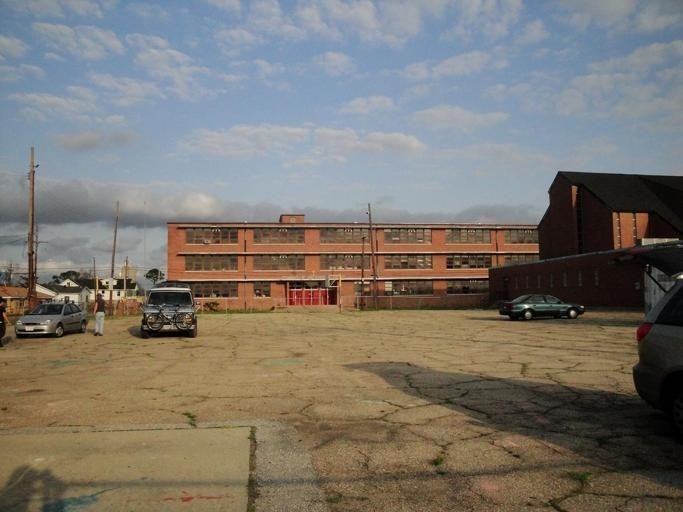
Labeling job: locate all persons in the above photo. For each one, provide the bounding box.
[0,296,11,347]
[92,293,108,336]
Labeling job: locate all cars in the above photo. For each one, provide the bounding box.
[141,283,197,337]
[15,303,88,337]
[499,294,585,320]
[633,272,683,439]
